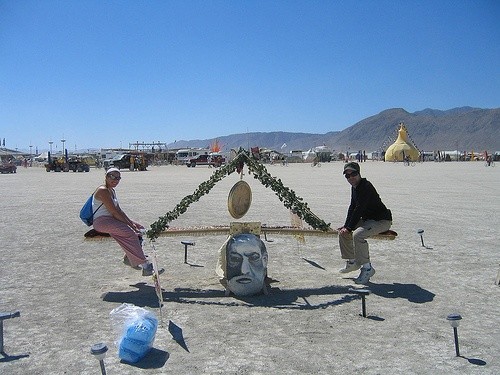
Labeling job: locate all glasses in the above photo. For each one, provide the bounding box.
[345,171,357,178]
[107,175,121,180]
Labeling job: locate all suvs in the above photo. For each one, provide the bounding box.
[103,154,148,171]
[184,154,225,167]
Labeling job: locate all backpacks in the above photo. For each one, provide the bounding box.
[79,185,106,226]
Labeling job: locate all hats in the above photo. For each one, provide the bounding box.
[106,168,120,175]
[343,162,360,174]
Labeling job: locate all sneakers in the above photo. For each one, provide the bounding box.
[123,256,142,270]
[339,261,360,273]
[142,263,165,276]
[355,263,375,284]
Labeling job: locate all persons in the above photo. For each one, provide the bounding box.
[404,154,411,165]
[128,154,147,170]
[312,157,317,165]
[268,154,287,166]
[92,168,164,277]
[486,156,493,166]
[339,162,392,285]
[206,154,222,168]
